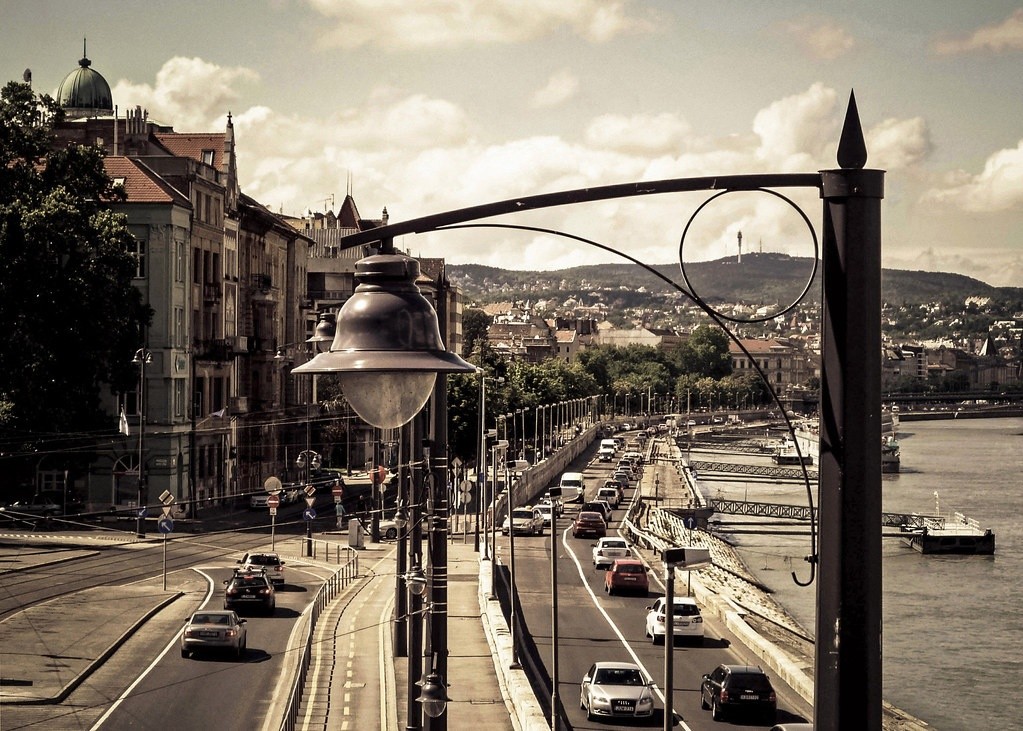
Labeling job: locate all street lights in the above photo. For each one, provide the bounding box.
[286,169,882,731]
[304,264,451,731]
[131,348,154,538]
[295,449,322,557]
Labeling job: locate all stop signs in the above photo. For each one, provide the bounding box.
[266,496,279,508]
[332,486,342,496]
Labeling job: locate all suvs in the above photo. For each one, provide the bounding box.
[236,552,285,585]
[223,566,275,612]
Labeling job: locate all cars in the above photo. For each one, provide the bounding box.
[598,414,739,489]
[4,492,60,517]
[769,722,814,731]
[502,472,624,538]
[645,597,704,645]
[250,482,305,507]
[580,662,656,721]
[603,558,650,596]
[701,664,777,721]
[366,512,429,539]
[181,611,247,657]
[590,537,633,569]
[41,489,86,511]
